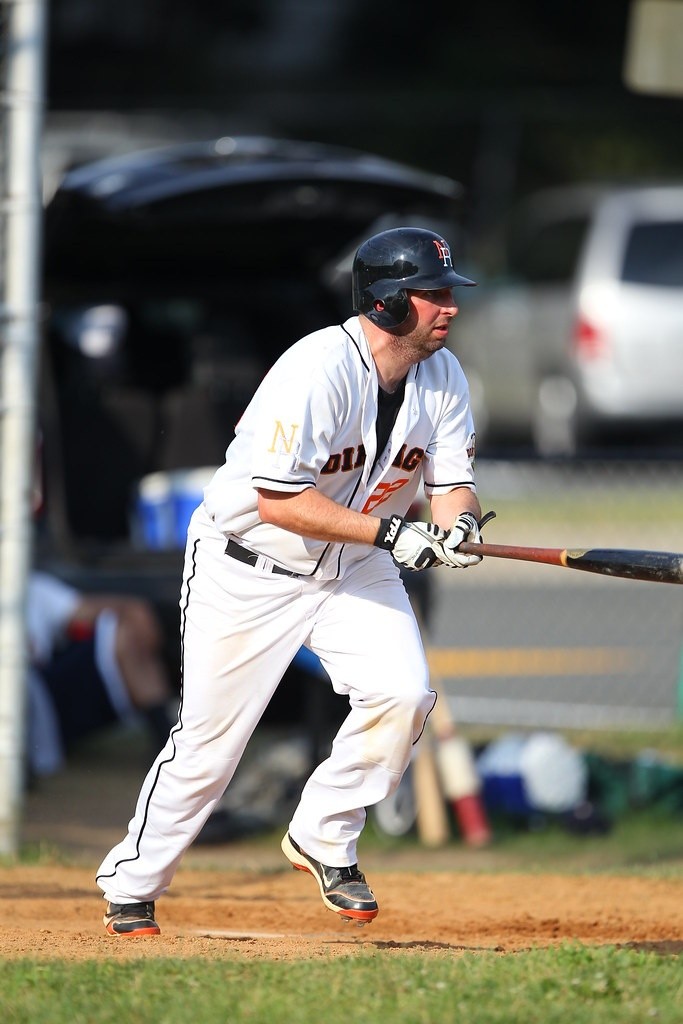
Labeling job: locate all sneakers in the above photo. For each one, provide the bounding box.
[280,831,379,928]
[102,899,160,937]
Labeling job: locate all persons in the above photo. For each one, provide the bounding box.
[93,226,496,938]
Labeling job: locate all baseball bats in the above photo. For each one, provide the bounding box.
[412,706,450,846]
[426,667,490,845]
[458,541,683,585]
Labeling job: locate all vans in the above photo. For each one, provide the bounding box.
[36,136,475,646]
[447,183,683,462]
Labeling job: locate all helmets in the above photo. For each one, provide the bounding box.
[351,226,476,328]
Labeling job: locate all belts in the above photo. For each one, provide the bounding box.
[225,539,302,578]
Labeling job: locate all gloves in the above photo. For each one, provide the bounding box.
[373,514,447,572]
[431,511,496,570]
